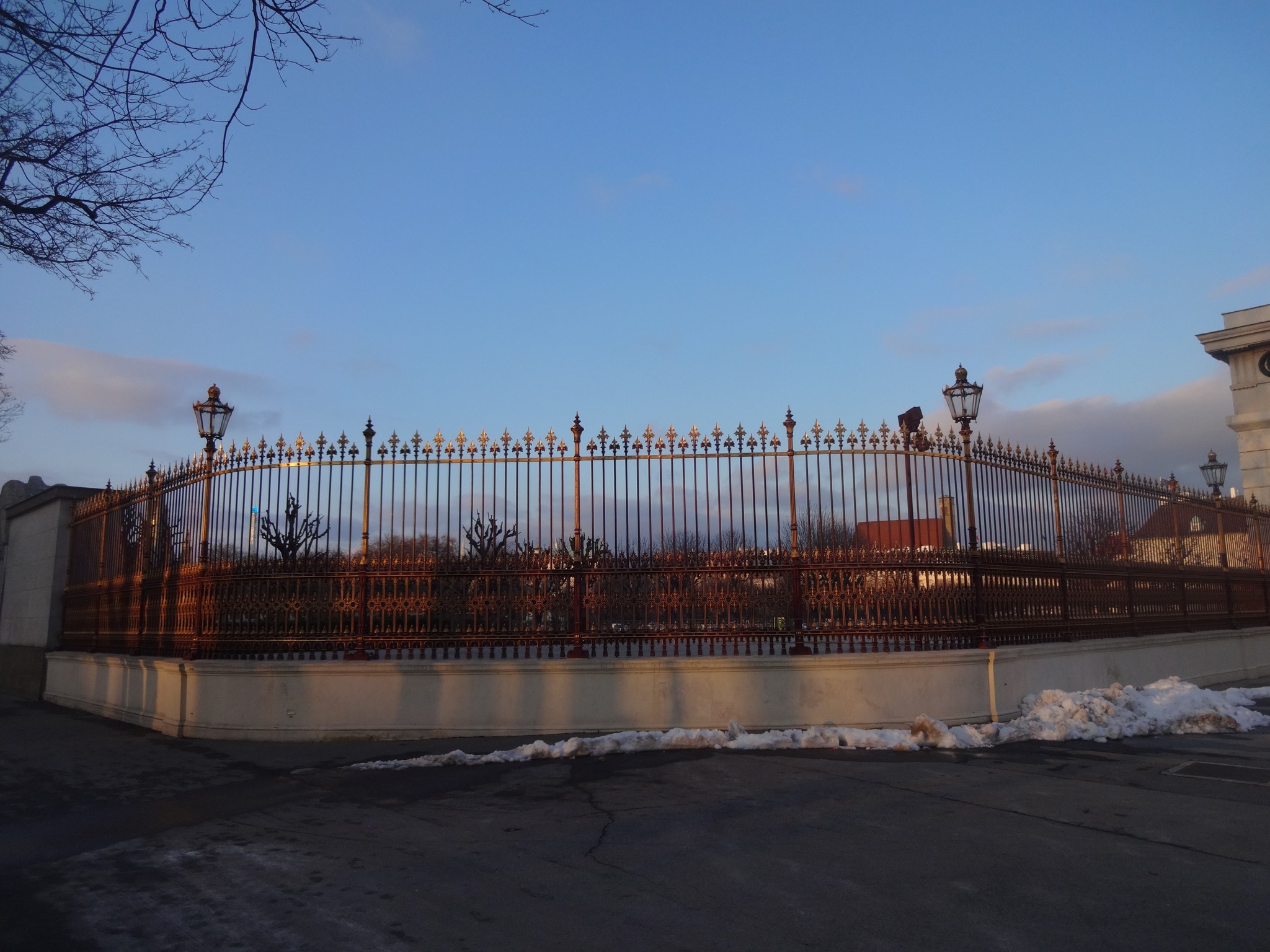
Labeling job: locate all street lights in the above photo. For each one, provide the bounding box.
[941,363,988,649]
[1198,448,1239,630]
[185,383,236,658]
